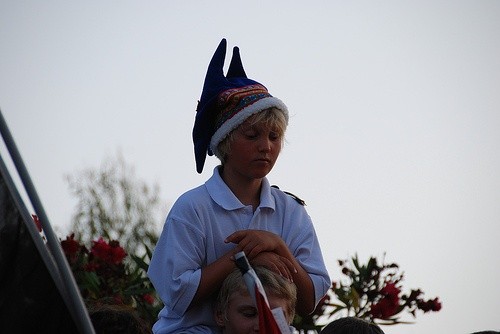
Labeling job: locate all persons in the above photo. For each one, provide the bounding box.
[145,90,332,334]
[215,265,297,334]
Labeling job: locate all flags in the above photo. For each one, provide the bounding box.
[234,252,292,334]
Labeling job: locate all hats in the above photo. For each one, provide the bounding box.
[192,38,289,174]
